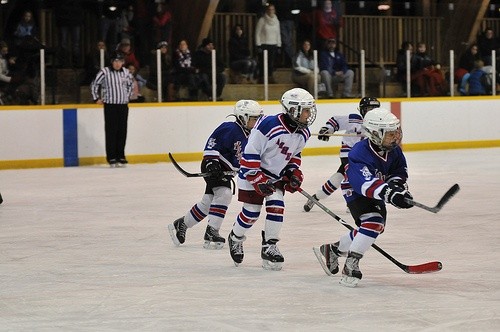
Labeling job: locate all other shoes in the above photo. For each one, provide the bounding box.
[340,93,355,98]
[328,95,334,98]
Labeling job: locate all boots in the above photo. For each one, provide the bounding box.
[168,216,188,246]
[119,158,128,167]
[203,225,225,250]
[303,194,319,212]
[313,241,348,276]
[261,231,285,270]
[240,73,258,84]
[228,229,246,267]
[339,250,362,287]
[106,157,117,168]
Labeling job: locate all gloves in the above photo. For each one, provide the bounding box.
[243,169,276,197]
[386,176,413,199]
[283,169,303,193]
[206,158,225,181]
[377,185,414,209]
[318,127,330,141]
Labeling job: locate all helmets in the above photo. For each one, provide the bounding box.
[360,108,403,150]
[280,88,317,130]
[360,96,380,119]
[234,99,263,129]
[110,53,125,64]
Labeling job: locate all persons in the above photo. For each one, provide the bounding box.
[303,96,381,213]
[169,99,267,250]
[228,87,317,271]
[12,11,40,60]
[302,0,346,74]
[291,40,322,101]
[266,0,296,68]
[317,37,356,98]
[0,41,39,106]
[254,3,282,87]
[444,67,473,97]
[312,108,415,288]
[396,41,435,97]
[89,50,136,165]
[456,44,493,95]
[226,23,259,85]
[476,27,500,85]
[0,53,20,98]
[412,41,450,97]
[80,0,227,103]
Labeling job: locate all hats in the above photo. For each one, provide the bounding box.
[156,40,168,49]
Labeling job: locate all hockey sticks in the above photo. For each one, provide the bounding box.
[404,184,460,213]
[296,187,442,274]
[310,133,367,137]
[169,152,235,177]
